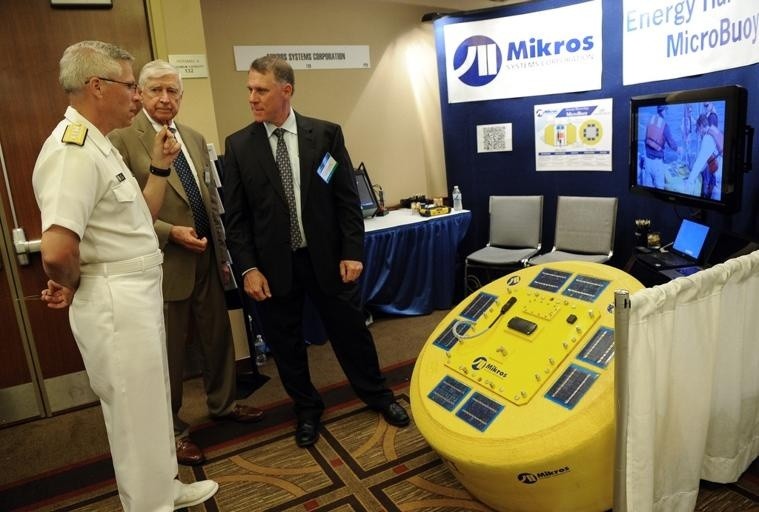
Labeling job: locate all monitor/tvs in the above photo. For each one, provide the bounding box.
[627,84,749,221]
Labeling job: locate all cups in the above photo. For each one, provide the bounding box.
[647,232,661,249]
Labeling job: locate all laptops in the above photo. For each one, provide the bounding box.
[637,218,711,268]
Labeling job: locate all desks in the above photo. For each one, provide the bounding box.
[240,204,474,365]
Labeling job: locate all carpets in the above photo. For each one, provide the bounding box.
[0,358,759,511]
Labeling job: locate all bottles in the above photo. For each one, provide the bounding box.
[452,185,462,210]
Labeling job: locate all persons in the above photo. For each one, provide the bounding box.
[105,60,266,465]
[33,40,219,512]
[224,56,409,447]
[642,100,724,201]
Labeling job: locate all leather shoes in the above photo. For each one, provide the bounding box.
[170,477,220,511]
[379,401,411,427]
[291,411,323,447]
[219,404,265,425]
[174,440,207,466]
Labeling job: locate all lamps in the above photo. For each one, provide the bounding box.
[419,11,470,23]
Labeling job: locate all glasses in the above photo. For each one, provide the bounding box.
[85,76,139,92]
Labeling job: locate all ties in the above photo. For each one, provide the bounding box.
[166,127,211,240]
[271,126,303,252]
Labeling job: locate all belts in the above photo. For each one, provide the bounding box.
[76,249,166,278]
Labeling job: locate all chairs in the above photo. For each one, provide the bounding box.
[525,193,618,268]
[463,194,545,301]
